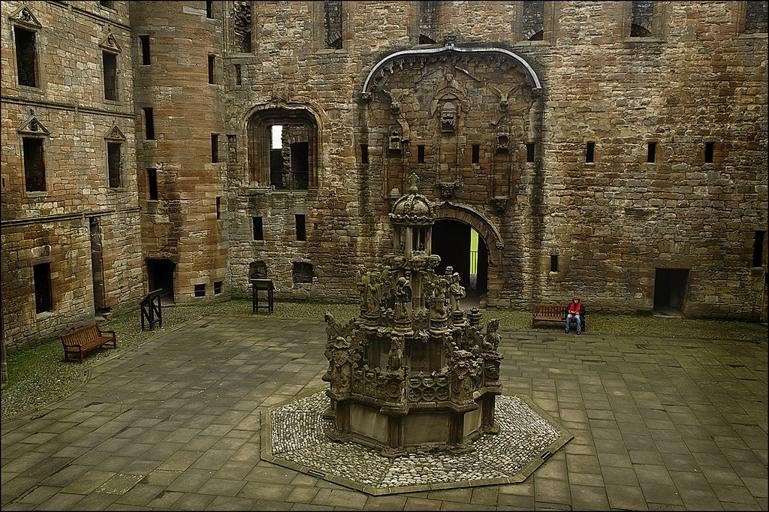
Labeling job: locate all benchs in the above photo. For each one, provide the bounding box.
[531,303,586,330]
[58,324,117,364]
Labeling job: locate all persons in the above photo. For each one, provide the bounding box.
[565,297,585,335]
